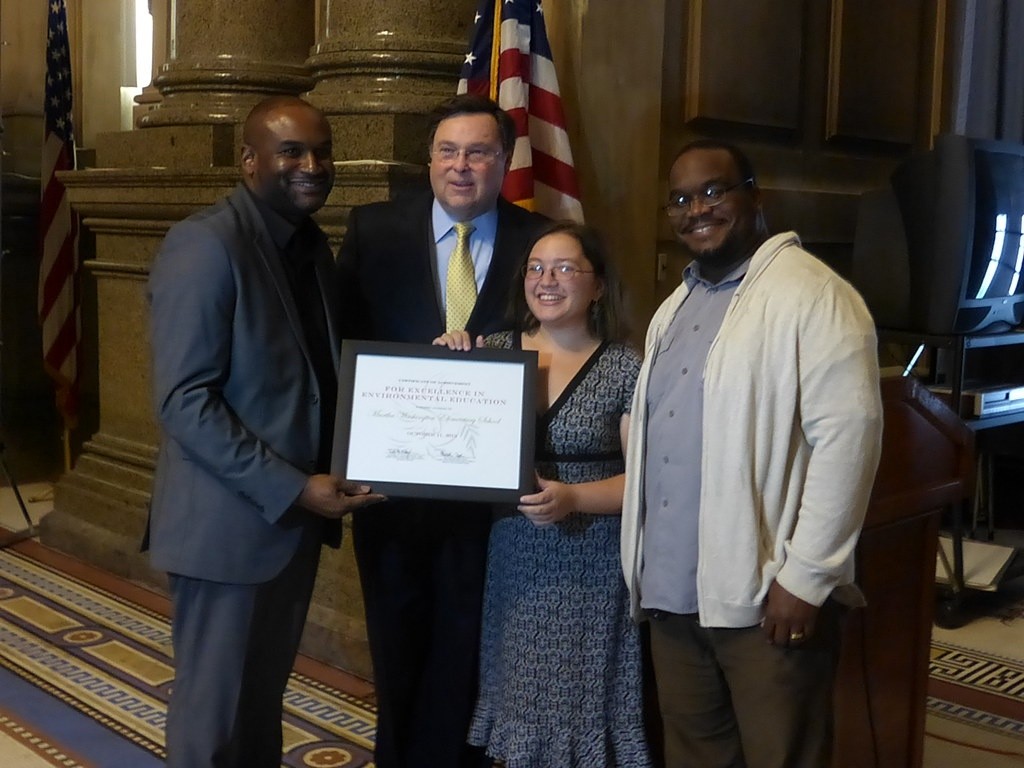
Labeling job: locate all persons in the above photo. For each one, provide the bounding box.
[620,136,884,768]
[432,220,645,768]
[327,93,562,768]
[137,96,386,768]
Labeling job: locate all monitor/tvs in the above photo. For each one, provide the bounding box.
[889,132,1024,338]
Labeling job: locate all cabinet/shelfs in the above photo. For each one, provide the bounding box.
[876,329,1024,629]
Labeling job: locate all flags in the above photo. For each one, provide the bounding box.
[456,0,588,227]
[37,0,82,430]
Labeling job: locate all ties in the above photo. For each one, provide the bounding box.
[446,222,477,334]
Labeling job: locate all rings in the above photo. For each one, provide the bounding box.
[790,634,803,639]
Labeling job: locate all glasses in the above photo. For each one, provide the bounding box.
[520,264,593,282]
[430,144,502,166]
[664,178,754,218]
